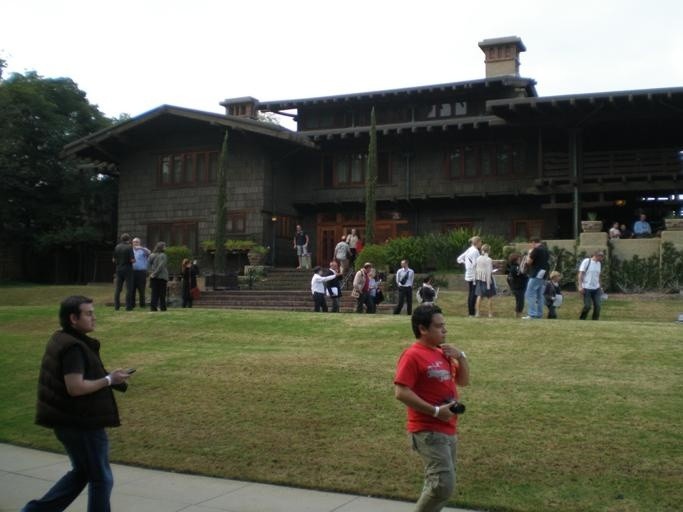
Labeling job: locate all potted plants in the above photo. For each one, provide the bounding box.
[662,211,682,231]
[202,237,253,274]
[580,211,602,232]
[165,246,192,308]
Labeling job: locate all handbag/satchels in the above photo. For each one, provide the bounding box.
[376,287,384,304]
[189,287,201,300]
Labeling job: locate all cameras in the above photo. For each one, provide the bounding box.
[107,372,129,392]
[443,398,465,413]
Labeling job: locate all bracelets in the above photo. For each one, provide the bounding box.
[433,406,439,418]
[105,376,111,386]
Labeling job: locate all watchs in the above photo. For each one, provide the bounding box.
[458,352,464,360]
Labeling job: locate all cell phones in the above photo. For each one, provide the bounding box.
[193,260,197,265]
[127,368,136,374]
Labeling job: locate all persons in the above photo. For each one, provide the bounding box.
[310,228,439,315]
[20,297,134,511]
[292,224,311,269]
[608,213,651,239]
[112,232,197,312]
[455,236,603,321]
[392,304,468,512]
